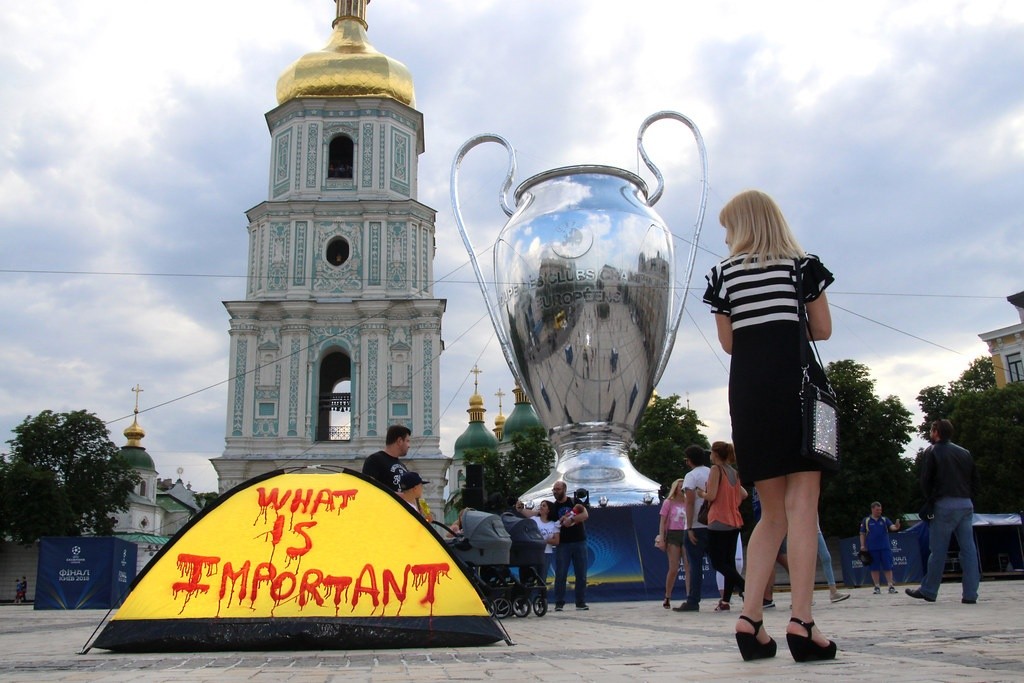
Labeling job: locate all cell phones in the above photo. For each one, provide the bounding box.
[895,519,899,528]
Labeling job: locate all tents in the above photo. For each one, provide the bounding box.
[76,466,518,656]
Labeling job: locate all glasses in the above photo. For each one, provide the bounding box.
[929,429,933,433]
[683,458,688,461]
[552,488,561,491]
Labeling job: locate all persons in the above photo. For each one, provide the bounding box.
[530,501,560,610]
[811,510,850,606]
[516,481,589,612]
[555,504,584,526]
[362,425,411,493]
[695,440,748,613]
[740,488,791,609]
[20,576,27,601]
[672,445,745,611]
[904,418,981,604]
[400,472,431,511]
[701,191,837,663]
[659,478,691,609]
[860,501,901,594]
[13,579,22,603]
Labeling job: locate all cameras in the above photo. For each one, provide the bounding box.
[518,503,524,509]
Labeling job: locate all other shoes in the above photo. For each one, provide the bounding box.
[961,598,976,604]
[576,605,589,610]
[538,602,551,613]
[555,606,562,611]
[905,588,935,602]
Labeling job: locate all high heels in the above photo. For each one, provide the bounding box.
[786,617,837,662]
[735,615,776,661]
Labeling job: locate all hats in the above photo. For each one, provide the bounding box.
[400,472,430,490]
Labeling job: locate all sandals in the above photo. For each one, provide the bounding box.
[663,593,671,609]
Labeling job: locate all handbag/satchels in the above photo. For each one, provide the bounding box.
[654,534,666,548]
[919,499,933,522]
[857,551,872,567]
[698,464,722,526]
[800,381,842,466]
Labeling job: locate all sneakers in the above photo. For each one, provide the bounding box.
[874,585,880,594]
[673,601,699,612]
[762,598,775,608]
[888,587,898,594]
[714,600,730,613]
[829,592,850,603]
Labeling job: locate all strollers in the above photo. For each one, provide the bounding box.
[433,508,525,619]
[501,511,550,618]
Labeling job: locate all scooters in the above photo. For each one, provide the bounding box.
[14,576,27,604]
